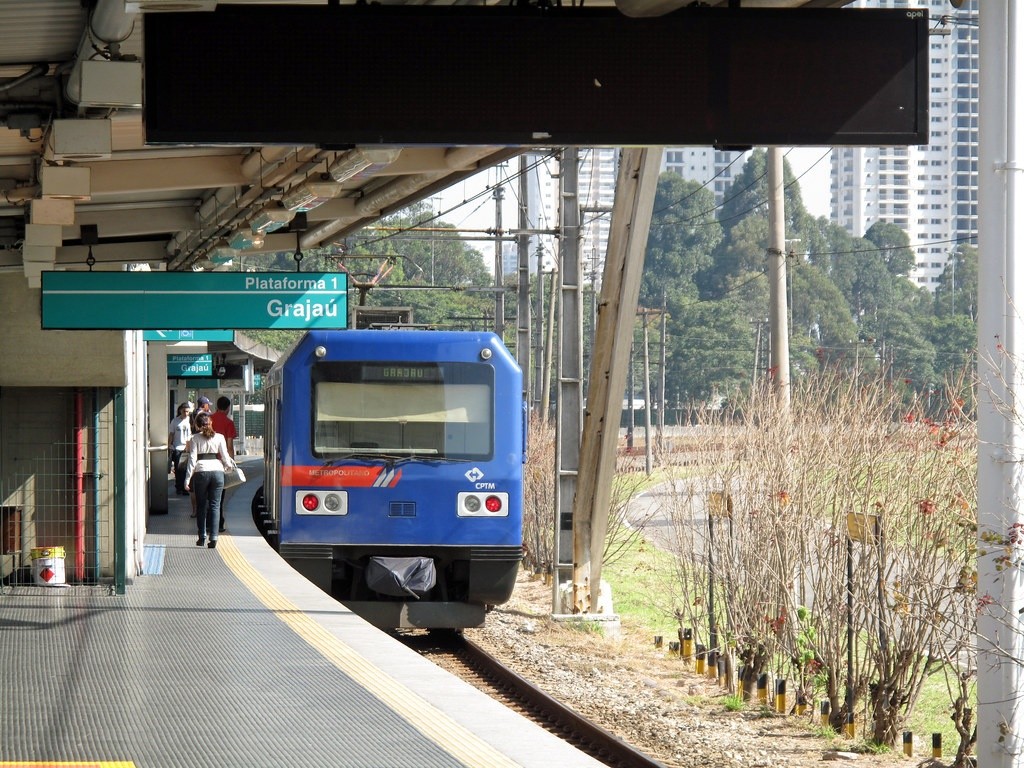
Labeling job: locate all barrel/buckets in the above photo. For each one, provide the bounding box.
[31,546,66,584]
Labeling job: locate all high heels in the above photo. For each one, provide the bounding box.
[197,537,206,546]
[207,541,216,548]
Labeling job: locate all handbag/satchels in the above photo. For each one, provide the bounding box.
[223,459,246,488]
[177,449,190,470]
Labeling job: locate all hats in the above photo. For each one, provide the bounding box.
[198,396,213,406]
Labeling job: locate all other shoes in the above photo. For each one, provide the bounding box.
[182,492,190,496]
[191,513,196,518]
[219,528,225,532]
[176,490,182,494]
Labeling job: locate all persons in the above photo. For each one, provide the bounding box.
[168,396,237,532]
[184,413,234,548]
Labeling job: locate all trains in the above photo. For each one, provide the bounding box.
[263,329,528,635]
